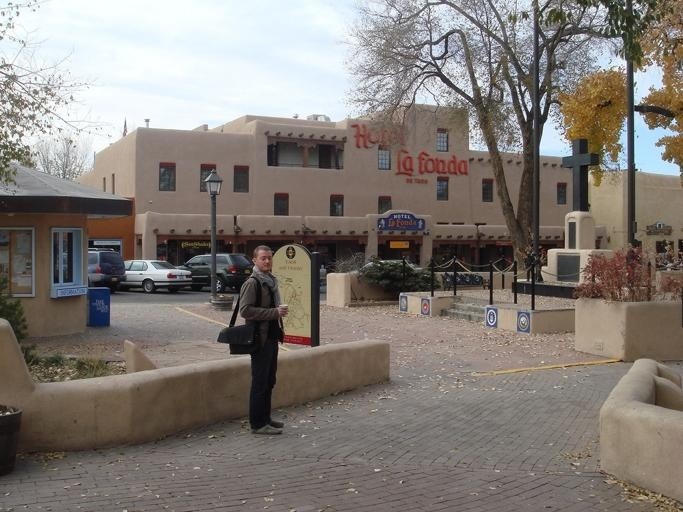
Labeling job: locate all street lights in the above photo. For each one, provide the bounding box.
[202,165,224,305]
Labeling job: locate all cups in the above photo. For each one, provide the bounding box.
[278,304,289,317]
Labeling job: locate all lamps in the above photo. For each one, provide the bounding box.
[301,223,311,236]
[233,226,240,234]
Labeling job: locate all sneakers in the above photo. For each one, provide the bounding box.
[267,421,284,428]
[251,424,283,435]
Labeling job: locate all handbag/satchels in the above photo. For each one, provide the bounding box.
[216,276,262,355]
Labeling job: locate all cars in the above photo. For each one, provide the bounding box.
[357,260,425,274]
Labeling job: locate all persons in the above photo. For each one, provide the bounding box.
[524,243,544,283]
[238,244,290,435]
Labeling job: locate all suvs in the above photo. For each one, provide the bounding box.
[53,246,253,294]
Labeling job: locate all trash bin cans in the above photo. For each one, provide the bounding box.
[87,286,110,327]
